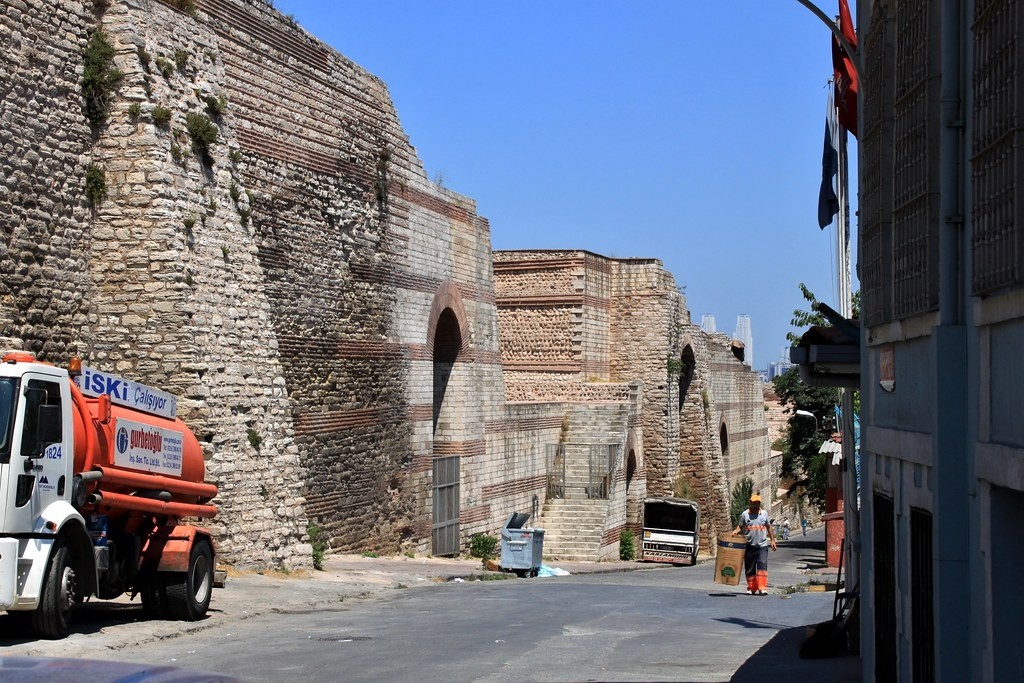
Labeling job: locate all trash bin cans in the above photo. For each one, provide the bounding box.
[501,511,546,577]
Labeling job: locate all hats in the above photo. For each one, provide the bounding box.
[751,495,762,503]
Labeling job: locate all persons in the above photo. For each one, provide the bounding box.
[731,495,777,596]
[800,514,809,536]
[781,516,791,540]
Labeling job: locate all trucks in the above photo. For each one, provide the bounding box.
[642,497,706,567]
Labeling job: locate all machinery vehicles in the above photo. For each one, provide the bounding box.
[0,350,230,642]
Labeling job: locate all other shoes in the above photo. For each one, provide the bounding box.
[759,590,767,595]
[746,590,752,595]
